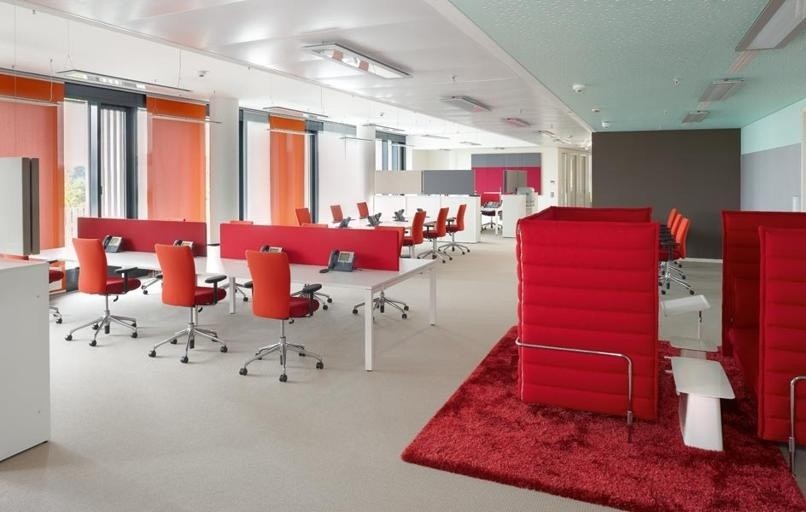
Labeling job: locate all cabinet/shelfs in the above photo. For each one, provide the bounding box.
[372,194,480,242]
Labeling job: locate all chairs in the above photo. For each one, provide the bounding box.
[3,254,65,324]
[65,238,142,347]
[237,249,324,382]
[513,204,806,455]
[423,208,453,264]
[295,200,439,235]
[39,218,207,296]
[149,243,227,363]
[174,220,410,322]
[481,192,505,233]
[402,211,426,260]
[438,204,471,255]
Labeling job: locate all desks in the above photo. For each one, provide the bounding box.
[175,256,437,372]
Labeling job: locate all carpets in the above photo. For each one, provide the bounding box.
[398,325,806,511]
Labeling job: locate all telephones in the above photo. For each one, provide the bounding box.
[368,212,382,227]
[484,201,496,208]
[102,234,123,253]
[340,216,352,228]
[259,244,285,253]
[328,248,355,272]
[392,209,405,221]
[173,239,195,251]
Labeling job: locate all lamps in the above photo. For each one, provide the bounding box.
[447,97,488,113]
[682,1,806,128]
[305,43,410,80]
[504,119,528,128]
[364,123,448,141]
[60,71,189,96]
[263,107,326,120]
